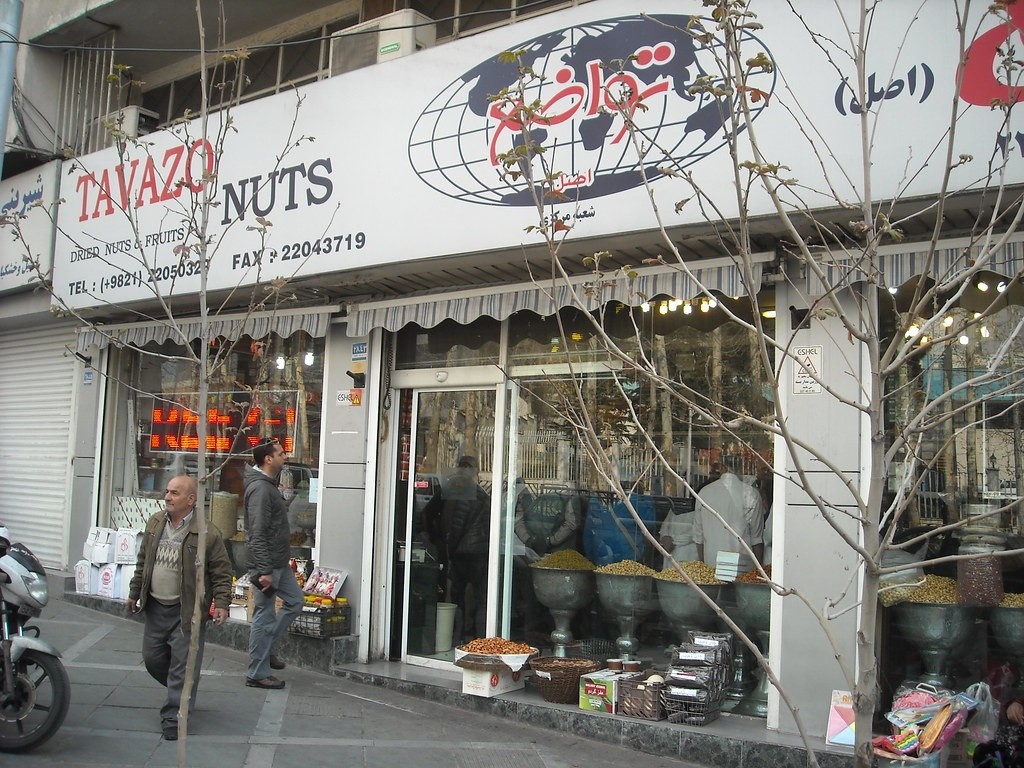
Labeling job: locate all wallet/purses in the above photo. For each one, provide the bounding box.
[249,570,281,599]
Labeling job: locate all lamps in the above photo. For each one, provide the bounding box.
[972,273,988,293]
[328,9,437,80]
[990,453,997,468]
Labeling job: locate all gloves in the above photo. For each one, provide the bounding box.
[525,535,550,557]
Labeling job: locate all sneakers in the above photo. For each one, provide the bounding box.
[270,655,285,670]
[244,674,285,689]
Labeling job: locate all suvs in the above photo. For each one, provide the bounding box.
[514,481,694,584]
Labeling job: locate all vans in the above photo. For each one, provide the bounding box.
[150,449,315,522]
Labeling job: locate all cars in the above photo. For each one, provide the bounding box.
[895,524,1024,593]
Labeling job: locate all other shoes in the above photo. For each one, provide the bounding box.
[164,727,178,740]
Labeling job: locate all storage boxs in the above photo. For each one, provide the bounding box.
[232,565,351,641]
[73,526,146,603]
[579,667,640,715]
[462,668,525,700]
[895,708,981,764]
[617,668,667,722]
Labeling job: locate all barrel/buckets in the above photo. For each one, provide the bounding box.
[436,602,458,651]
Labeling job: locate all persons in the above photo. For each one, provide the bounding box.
[418,456,489,642]
[241,438,305,689]
[514,450,772,655]
[126,476,233,741]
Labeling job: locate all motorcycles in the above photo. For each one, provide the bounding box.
[0,524,71,753]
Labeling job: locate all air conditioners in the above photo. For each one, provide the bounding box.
[86,104,159,155]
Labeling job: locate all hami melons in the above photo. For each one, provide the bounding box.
[622,675,665,714]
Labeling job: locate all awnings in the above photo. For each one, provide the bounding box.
[74,303,342,355]
[345,252,777,339]
[805,225,1024,298]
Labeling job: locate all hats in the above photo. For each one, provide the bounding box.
[459,456,478,469]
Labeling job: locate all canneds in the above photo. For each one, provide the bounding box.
[304,595,347,623]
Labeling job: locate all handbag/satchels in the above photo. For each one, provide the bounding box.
[961,682,1003,744]
